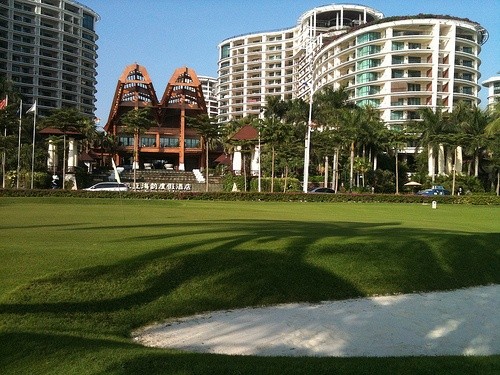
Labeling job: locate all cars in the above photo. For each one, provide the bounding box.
[419,190,444,195]
[83,181,130,191]
[307,187,335,194]
